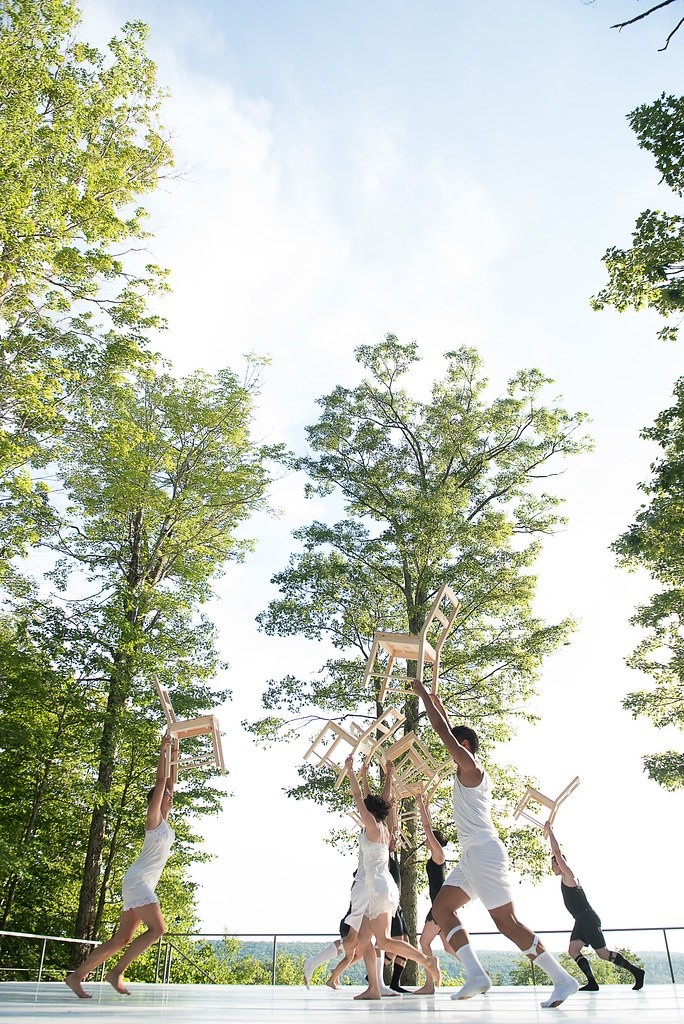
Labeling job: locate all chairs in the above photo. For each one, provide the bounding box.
[351,721,438,800]
[363,583,462,703]
[393,751,457,808]
[153,678,227,783]
[303,706,407,795]
[512,775,580,839]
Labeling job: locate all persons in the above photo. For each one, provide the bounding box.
[64,730,178,998]
[543,821,645,991]
[302,754,440,999]
[411,678,579,1008]
[412,791,489,995]
